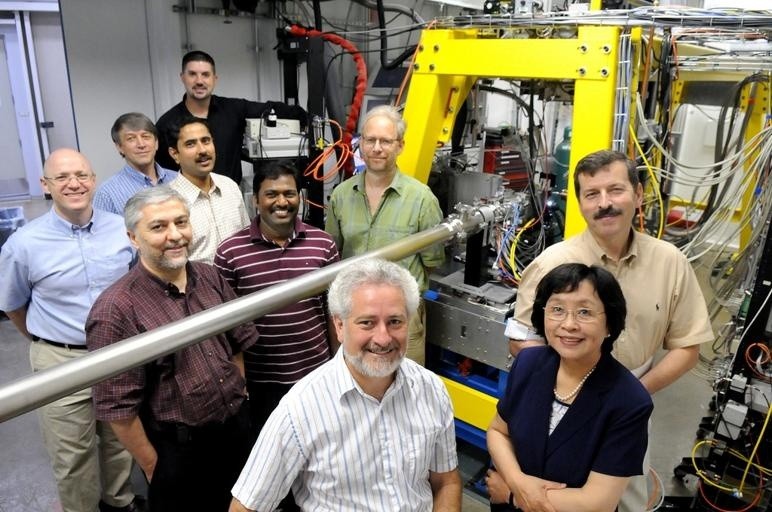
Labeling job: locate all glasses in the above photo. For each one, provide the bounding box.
[362,135,399,150]
[543,304,605,324]
[45,172,92,185]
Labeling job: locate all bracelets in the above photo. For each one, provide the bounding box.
[508,492,515,506]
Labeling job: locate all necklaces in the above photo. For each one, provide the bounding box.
[554,366,596,402]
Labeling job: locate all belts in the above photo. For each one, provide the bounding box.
[31,334,86,350]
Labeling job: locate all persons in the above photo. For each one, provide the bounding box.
[214,164,340,512]
[484,261,655,512]
[165,115,251,265]
[92,111,177,221]
[228,257,463,512]
[503,148,715,511]
[325,103,447,366]
[84,184,260,512]
[0,148,148,512]
[155,50,303,184]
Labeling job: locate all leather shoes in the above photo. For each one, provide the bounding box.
[99,494,149,511]
